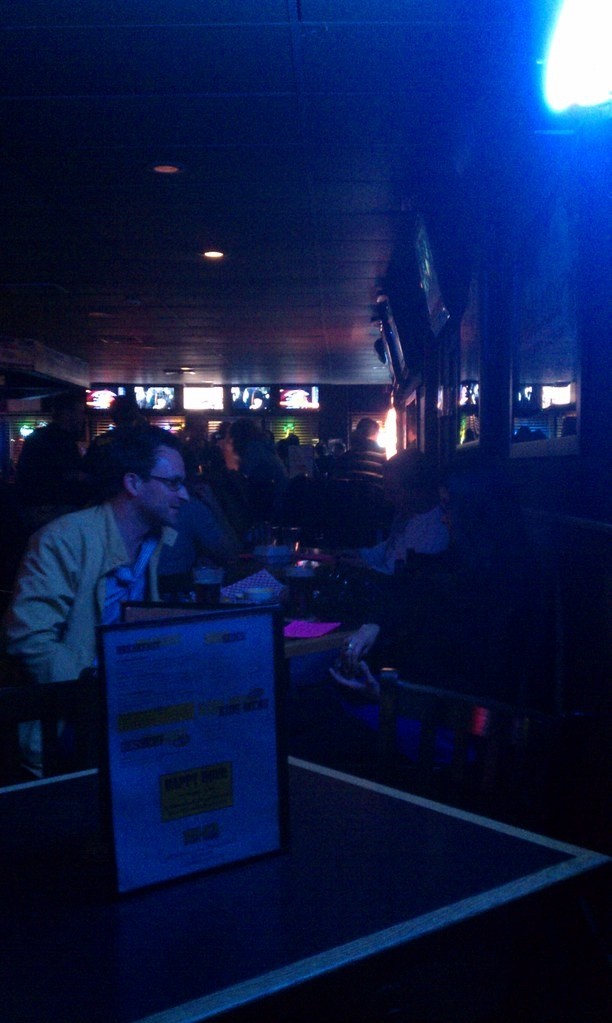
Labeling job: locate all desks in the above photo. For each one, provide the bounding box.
[0,755,612,1023]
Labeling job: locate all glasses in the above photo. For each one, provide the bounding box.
[149,475,192,493]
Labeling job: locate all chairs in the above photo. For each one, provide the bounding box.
[293,664,590,1023]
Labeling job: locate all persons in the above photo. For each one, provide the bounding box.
[0,380,612,780]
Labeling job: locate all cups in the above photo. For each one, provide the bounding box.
[286,567,315,619]
[193,566,222,602]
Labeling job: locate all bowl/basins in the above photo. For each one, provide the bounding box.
[245,586,273,602]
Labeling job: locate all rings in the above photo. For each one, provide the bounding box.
[343,644,354,650]
